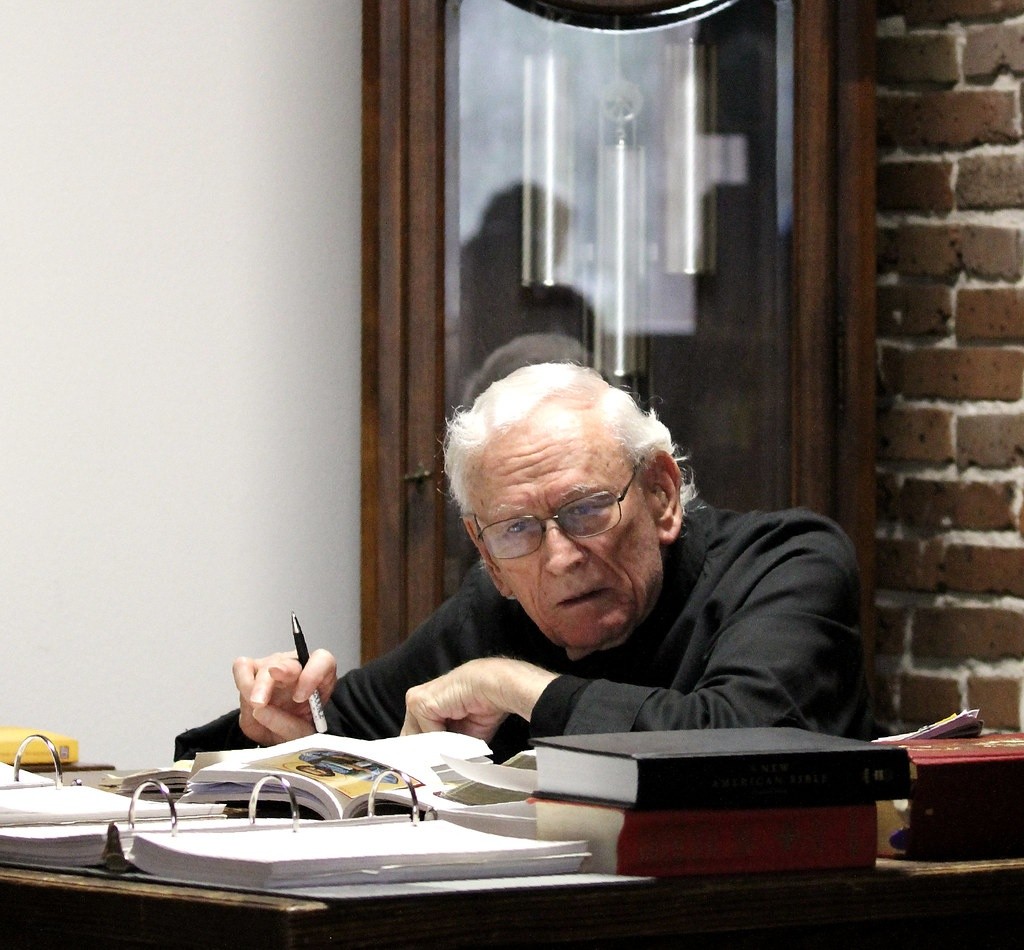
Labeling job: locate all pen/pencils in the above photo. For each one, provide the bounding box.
[290,610,329,735]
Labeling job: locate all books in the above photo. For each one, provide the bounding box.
[0,726,1024,889]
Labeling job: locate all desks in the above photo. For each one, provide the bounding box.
[0,769,1024,950]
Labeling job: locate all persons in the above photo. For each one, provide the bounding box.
[173,360,868,772]
[296,750,399,785]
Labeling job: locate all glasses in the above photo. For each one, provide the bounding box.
[474,458,644,560]
